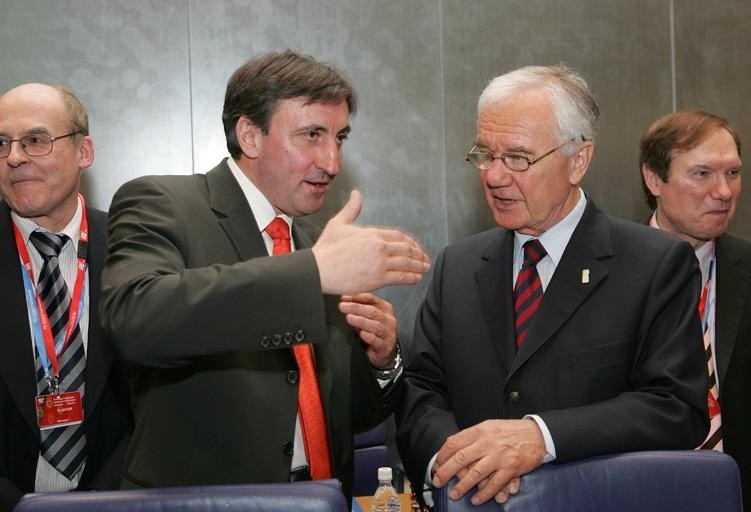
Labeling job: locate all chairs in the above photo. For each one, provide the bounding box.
[440,448,745,511]
[10,477,345,511]
[354,418,395,487]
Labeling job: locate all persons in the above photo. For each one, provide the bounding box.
[392,64,712,505]
[0,81,135,492]
[93,47,430,512]
[629,110,750,510]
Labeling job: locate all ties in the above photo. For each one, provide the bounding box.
[265,217,333,481]
[694,249,724,453]
[513,239,548,353]
[29,229,88,481]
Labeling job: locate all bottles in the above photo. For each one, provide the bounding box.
[371,466,401,512]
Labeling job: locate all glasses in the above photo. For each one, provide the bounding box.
[0,131,84,159]
[466,139,575,172]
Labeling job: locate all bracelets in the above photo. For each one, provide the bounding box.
[370,341,405,380]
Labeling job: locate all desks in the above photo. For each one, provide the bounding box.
[356,494,414,512]
